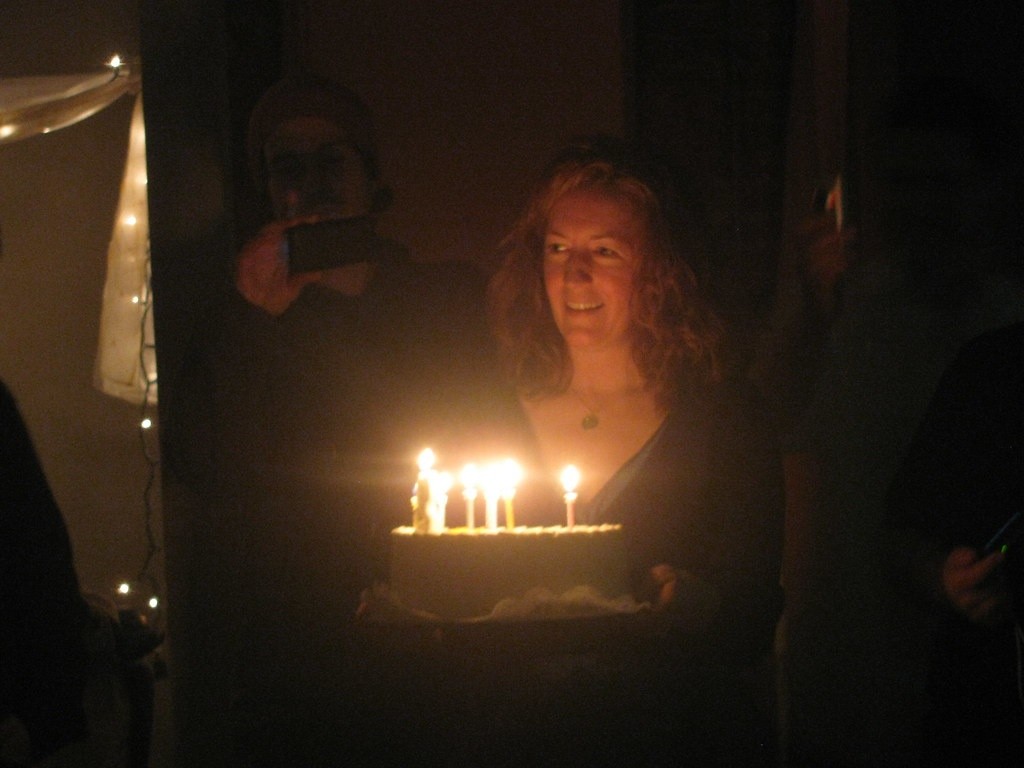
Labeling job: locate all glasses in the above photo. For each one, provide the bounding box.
[267,139,357,183]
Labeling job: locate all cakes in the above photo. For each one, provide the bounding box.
[381,524,632,622]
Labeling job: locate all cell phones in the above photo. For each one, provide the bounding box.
[282,216,374,274]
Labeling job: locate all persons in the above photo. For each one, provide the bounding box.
[172,75,486,618]
[351,133,739,622]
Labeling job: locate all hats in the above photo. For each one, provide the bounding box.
[245,69,378,212]
